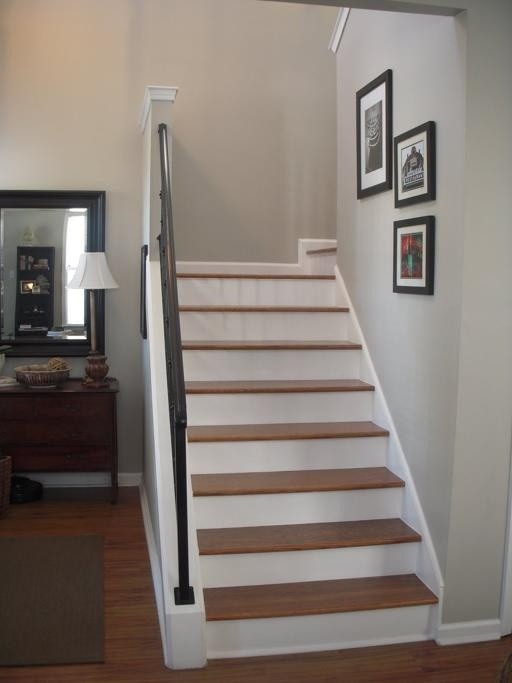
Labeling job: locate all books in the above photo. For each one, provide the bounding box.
[0,375,17,384]
[0,382,21,388]
[47,323,87,337]
[18,321,49,332]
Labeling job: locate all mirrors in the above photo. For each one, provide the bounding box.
[1,187,107,359]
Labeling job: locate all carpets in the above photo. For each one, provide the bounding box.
[0,532,110,669]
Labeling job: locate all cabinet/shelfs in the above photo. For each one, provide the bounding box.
[0,376,122,509]
[12,244,56,333]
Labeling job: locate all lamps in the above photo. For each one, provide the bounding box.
[67,251,120,388]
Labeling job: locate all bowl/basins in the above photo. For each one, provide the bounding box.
[34,264,49,269]
[15,364,71,389]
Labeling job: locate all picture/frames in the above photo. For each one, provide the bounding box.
[392,214,435,295]
[390,119,436,209]
[354,67,393,200]
